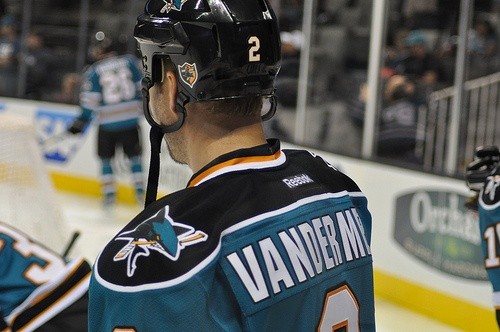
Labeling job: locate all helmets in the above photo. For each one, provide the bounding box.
[133,0,282,103]
[90,31,118,54]
[466,146,500,192]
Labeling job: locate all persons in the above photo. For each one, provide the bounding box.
[87,0,376,332]
[1,0,500,170]
[66,29,146,209]
